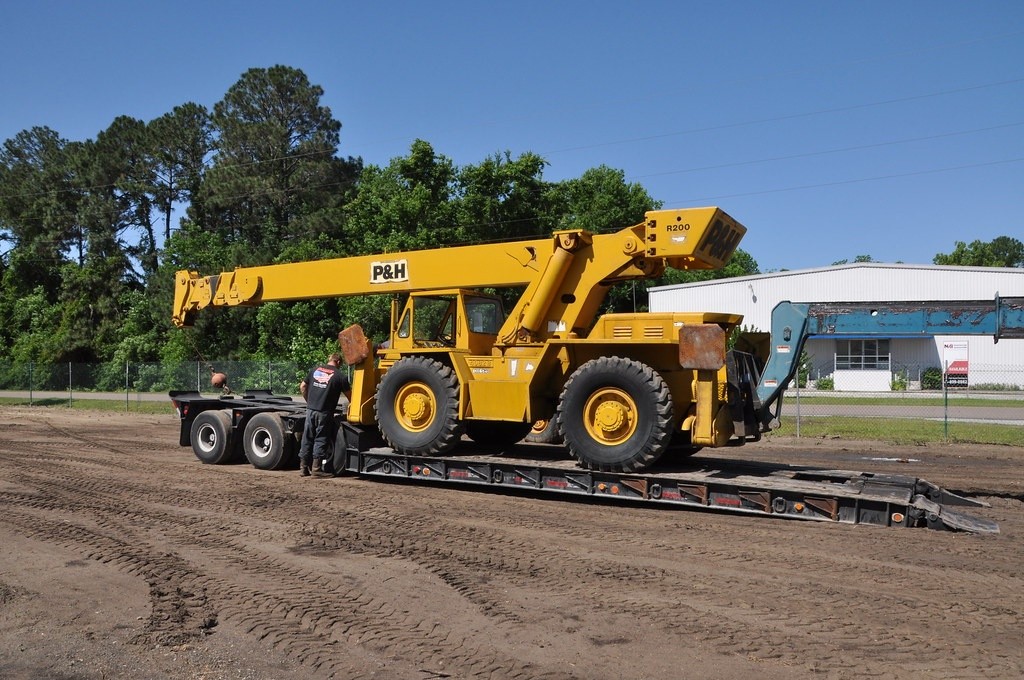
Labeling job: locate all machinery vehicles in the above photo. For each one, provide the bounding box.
[165,205,1024,471]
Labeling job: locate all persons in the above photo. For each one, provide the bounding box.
[297,353,352,476]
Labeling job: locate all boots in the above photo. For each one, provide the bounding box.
[300,460,310,476]
[311,459,333,478]
